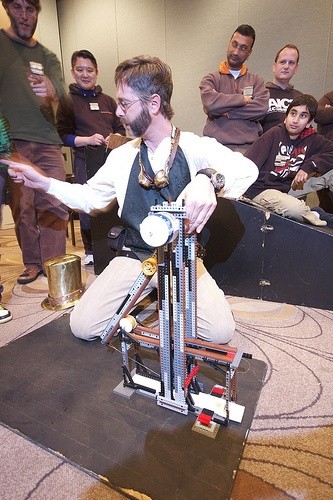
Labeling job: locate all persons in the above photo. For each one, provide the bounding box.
[0,2,71,284]
[258,45,304,138]
[56,49,126,266]
[199,24,270,155]
[243,94,333,228]
[0,55,260,341]
[313,90,333,215]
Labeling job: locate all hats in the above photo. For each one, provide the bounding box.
[41,254,87,311]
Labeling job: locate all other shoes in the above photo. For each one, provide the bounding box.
[311,207,333,229]
[17,267,43,284]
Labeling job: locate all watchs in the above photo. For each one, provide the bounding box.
[195,169,225,196]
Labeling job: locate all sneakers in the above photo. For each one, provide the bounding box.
[0,306,13,324]
[84,254,94,265]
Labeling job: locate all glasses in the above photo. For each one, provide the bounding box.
[9,3,37,17]
[116,100,141,111]
